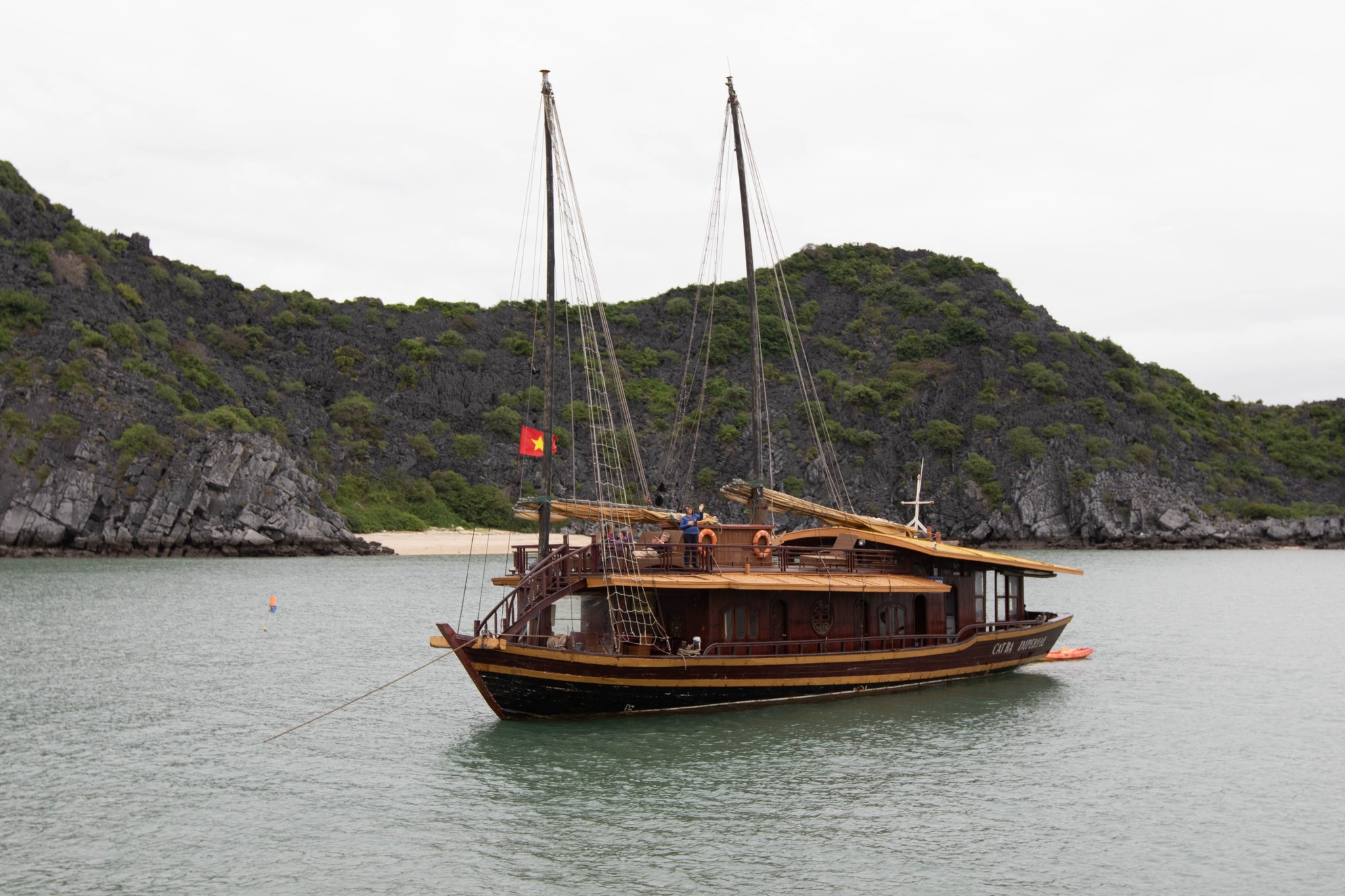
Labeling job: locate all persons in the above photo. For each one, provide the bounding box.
[628,532,672,560]
[680,503,705,568]
[600,524,633,572]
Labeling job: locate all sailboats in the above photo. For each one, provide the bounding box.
[431,68,1088,720]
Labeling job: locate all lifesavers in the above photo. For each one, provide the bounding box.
[698,528,718,558]
[752,529,772,558]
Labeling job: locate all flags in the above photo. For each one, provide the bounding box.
[519,425,558,456]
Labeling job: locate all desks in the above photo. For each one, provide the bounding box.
[598,633,639,651]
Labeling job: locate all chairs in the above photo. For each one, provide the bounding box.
[584,632,611,654]
[799,533,859,574]
[554,530,684,570]
[623,634,654,655]
